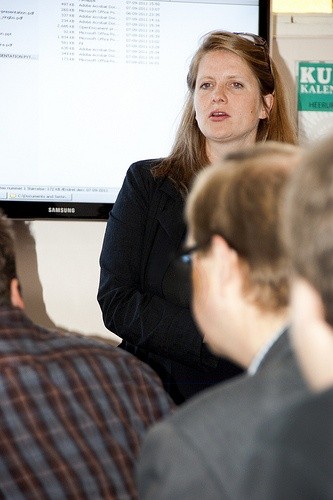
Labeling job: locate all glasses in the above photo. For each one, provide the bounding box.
[232,30,268,49]
[173,235,210,270]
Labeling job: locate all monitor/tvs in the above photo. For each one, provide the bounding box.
[1,0,270,219]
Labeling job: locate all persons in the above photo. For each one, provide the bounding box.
[137,133,333,500]
[97,30,299,406]
[0,212,179,500]
